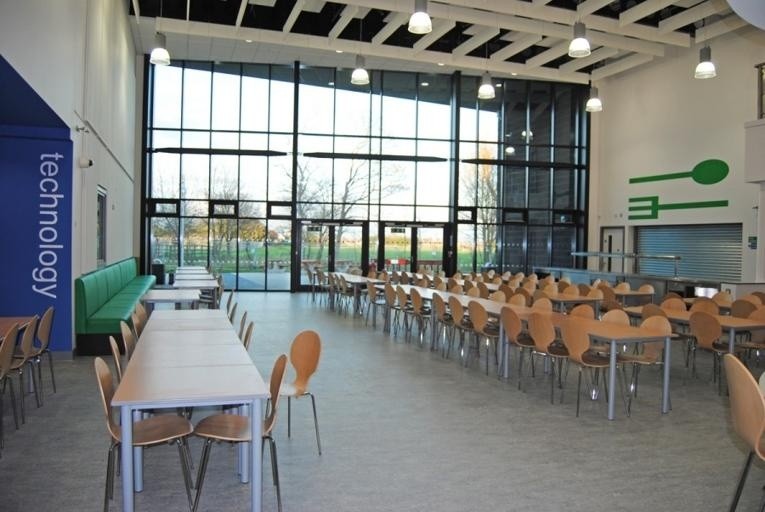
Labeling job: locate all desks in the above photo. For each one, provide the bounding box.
[2,316,37,341]
[111,309,273,511]
[174,266,221,310]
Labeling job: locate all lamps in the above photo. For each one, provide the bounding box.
[476,42,496,100]
[350,6,370,85]
[568,0,591,58]
[408,0,432,34]
[585,64,603,113]
[694,18,716,79]
[149,0,171,66]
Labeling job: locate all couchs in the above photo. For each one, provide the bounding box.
[74,258,157,355]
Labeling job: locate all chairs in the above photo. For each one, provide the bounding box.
[1,325,20,448]
[226,288,233,313]
[464,301,498,376]
[560,317,630,418]
[305,258,762,358]
[94,357,195,511]
[9,314,40,424]
[193,353,288,511]
[723,353,764,510]
[736,310,764,369]
[12,305,54,406]
[501,306,537,390]
[528,312,571,404]
[237,311,248,339]
[108,336,195,425]
[243,321,254,350]
[689,311,747,397]
[228,302,238,326]
[624,317,674,413]
[264,329,322,455]
